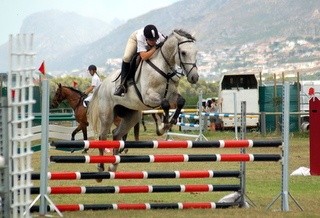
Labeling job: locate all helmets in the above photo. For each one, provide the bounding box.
[88,65,97,70]
[144,24,162,41]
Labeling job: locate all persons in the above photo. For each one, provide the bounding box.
[113,25,165,95]
[84,65,101,107]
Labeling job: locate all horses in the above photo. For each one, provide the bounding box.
[51,83,140,155]
[90,30,200,182]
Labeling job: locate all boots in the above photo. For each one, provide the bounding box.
[85,101,89,106]
[114,61,130,96]
